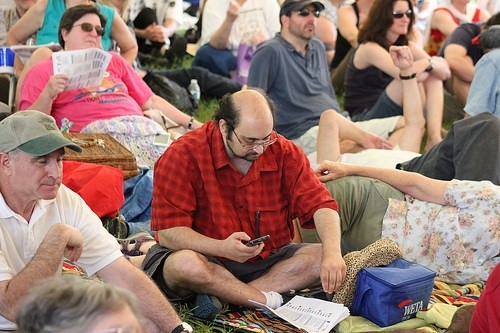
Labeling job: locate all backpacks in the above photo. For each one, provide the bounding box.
[142,71,199,115]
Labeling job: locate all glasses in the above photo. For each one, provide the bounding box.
[72,22,104,35]
[292,8,320,18]
[92,321,141,333]
[393,9,412,18]
[229,126,279,150]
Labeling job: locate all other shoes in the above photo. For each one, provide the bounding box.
[166,37,186,69]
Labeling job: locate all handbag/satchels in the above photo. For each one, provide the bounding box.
[350,258,436,326]
[230,33,258,85]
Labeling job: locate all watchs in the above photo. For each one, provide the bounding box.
[424,58,435,72]
[170,322,193,333]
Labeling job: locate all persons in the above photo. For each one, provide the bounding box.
[0,0,500,333]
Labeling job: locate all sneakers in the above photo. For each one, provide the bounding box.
[191,294,222,319]
[283,290,307,308]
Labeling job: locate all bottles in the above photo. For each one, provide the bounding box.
[188,79,200,104]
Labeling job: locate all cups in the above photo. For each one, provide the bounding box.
[0,48,15,73]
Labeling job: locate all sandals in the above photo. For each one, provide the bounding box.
[115,229,155,257]
[104,215,129,238]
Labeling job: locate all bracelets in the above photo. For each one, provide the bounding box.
[188,117,194,130]
[399,71,416,80]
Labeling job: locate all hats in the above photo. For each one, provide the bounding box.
[280,0,325,12]
[332,239,405,311]
[0,109,83,155]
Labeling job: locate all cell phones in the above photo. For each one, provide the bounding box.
[246,234,270,247]
